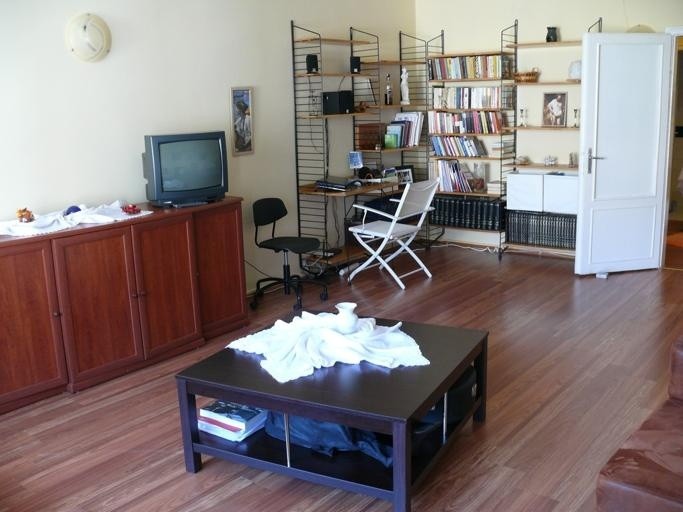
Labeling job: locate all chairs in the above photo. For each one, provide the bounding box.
[346,176,442,290]
[250,197,329,310]
[596,340,683,512]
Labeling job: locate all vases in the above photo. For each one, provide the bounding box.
[545,26,558,41]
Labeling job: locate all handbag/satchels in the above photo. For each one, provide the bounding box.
[265,409,394,468]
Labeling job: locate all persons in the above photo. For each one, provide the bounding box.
[399,67,411,106]
[546,94,563,126]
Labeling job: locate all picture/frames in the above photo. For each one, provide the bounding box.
[541,92,568,128]
[394,165,415,188]
[229,87,254,157]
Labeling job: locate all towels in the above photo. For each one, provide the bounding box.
[225,311,430,383]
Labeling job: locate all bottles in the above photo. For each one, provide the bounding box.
[383,73,392,105]
[546,26,557,42]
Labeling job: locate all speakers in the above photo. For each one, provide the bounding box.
[306,54,318,73]
[322,91,354,115]
[350,57,361,74]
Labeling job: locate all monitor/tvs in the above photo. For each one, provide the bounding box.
[142,131,229,209]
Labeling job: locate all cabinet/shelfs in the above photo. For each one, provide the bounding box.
[0,240,69,415]
[192,195,251,339]
[50,212,205,394]
[354,62,430,254]
[428,51,516,248]
[508,39,585,176]
[290,20,398,279]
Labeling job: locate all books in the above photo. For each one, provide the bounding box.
[383,111,425,150]
[317,175,354,189]
[428,54,516,232]
[196,398,269,442]
[505,208,576,253]
[317,184,355,192]
